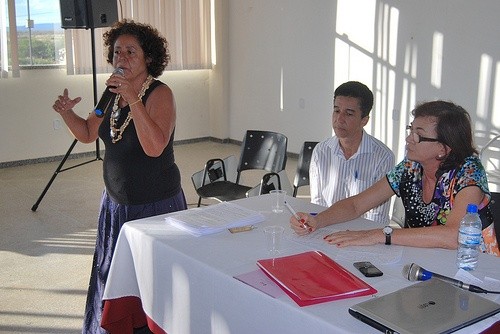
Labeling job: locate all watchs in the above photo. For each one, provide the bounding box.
[383,226,392,245]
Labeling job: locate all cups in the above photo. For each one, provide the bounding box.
[270,190,286,213]
[265,227,284,254]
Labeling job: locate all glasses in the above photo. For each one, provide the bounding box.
[406,125,453,148]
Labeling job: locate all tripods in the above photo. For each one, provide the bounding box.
[31,28,104,213]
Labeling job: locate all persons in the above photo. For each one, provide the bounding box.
[309,81,395,225]
[289,101,500,257]
[53,20,188,334]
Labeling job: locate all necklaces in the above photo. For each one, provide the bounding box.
[110,75,154,143]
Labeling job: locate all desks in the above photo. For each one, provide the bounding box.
[99,191,500,334]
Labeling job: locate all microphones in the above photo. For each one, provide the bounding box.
[95,67,125,116]
[401,263,487,293]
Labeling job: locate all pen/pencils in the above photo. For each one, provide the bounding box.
[284,201,307,228]
[354,171,358,181]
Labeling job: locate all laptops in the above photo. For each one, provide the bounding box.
[348,277,500,334]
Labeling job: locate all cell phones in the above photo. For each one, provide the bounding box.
[353,261,383,277]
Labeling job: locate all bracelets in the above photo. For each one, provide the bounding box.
[129,100,140,107]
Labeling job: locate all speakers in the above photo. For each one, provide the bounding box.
[58,0,118,30]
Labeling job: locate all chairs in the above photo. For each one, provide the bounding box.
[197,129,288,208]
[292,141,321,198]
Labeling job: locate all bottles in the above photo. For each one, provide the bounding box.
[456,204,483,271]
